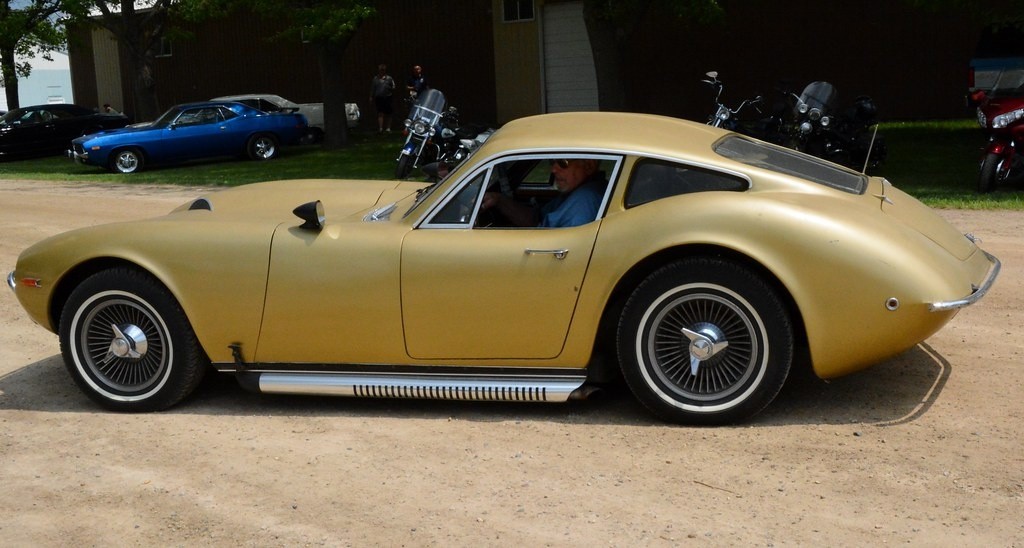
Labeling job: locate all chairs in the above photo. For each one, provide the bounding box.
[637,164,676,195]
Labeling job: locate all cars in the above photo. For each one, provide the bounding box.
[71,100,309,175]
[0,102,132,163]
[128,94,325,148]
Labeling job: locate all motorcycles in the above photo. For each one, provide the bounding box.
[965,66,1024,197]
[770,80,888,173]
[699,71,792,139]
[395,87,501,181]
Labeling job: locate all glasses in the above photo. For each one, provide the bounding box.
[548,159,575,169]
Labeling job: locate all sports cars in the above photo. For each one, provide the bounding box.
[8,111,1002,428]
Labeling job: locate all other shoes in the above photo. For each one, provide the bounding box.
[386,128,392,135]
[377,128,383,135]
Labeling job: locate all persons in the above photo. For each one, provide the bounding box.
[369,64,395,132]
[103,103,118,114]
[42,111,51,122]
[471,159,609,227]
[406,66,428,99]
